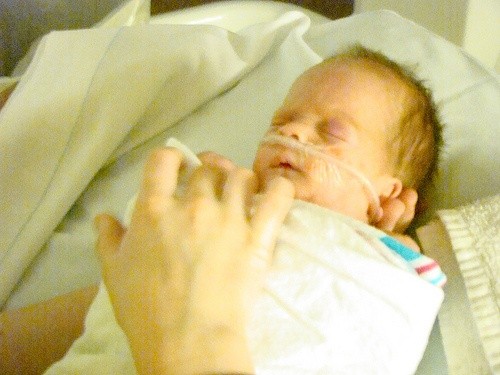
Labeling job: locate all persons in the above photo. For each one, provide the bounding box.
[45,40,448,375]
[1,146,423,374]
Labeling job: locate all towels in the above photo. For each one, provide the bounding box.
[431,190,499,375]
[39,135,449,375]
